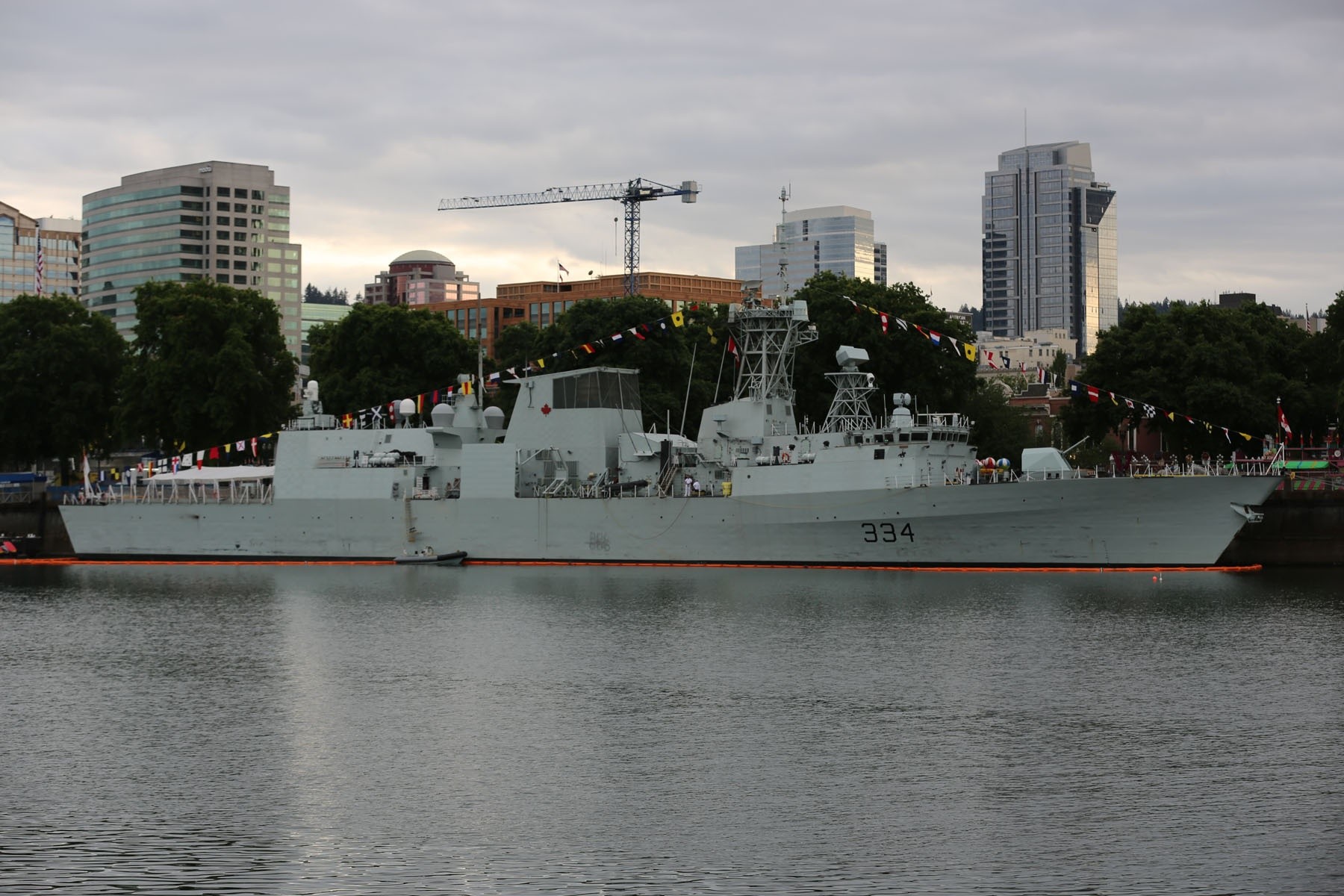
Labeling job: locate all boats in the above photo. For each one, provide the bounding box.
[394,550,467,567]
[54,180,1288,578]
[0,536,47,553]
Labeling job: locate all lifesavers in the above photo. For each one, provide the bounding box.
[782,453,790,463]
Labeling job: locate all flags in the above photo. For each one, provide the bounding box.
[627,305,698,340]
[137,454,192,478]
[566,333,623,360]
[98,466,131,483]
[1202,421,1252,444]
[490,352,562,385]
[929,330,1010,370]
[417,381,472,413]
[1020,362,1099,403]
[36,235,43,293]
[1108,392,1195,425]
[1307,310,1311,333]
[559,264,569,274]
[342,402,394,428]
[83,456,90,496]
[197,433,273,470]
[727,336,739,362]
[844,296,929,340]
[1278,405,1292,440]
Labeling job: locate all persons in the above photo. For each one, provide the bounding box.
[445,478,460,497]
[684,474,692,496]
[693,480,700,492]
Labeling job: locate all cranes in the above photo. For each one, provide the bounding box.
[437,176,703,303]
